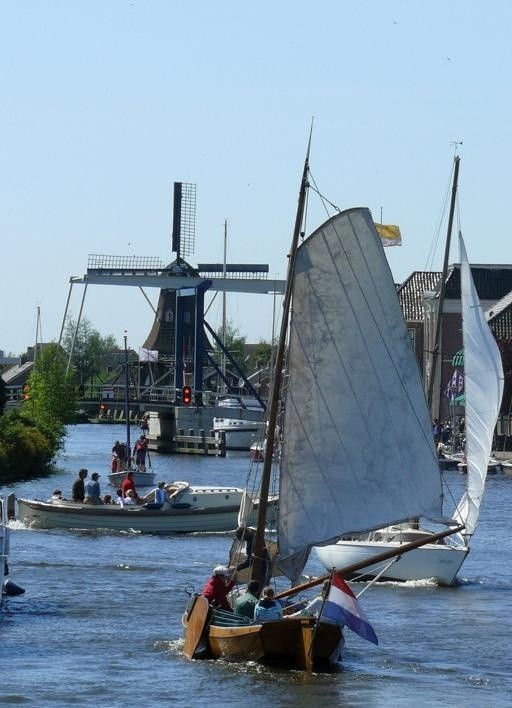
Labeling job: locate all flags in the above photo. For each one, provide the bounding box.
[321,567,381,645]
[375,220,402,249]
[442,367,466,404]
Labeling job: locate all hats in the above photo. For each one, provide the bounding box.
[92,472,101,478]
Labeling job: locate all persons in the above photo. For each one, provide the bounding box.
[232,579,263,620]
[433,415,467,464]
[102,494,112,504]
[110,440,136,473]
[124,488,137,504]
[121,471,142,505]
[227,525,270,596]
[200,564,239,612]
[154,481,169,503]
[52,488,68,500]
[140,415,149,434]
[253,585,283,620]
[132,434,149,472]
[84,471,102,504]
[284,577,331,619]
[70,467,88,502]
[116,487,124,510]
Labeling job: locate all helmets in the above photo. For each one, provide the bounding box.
[213,566,230,577]
